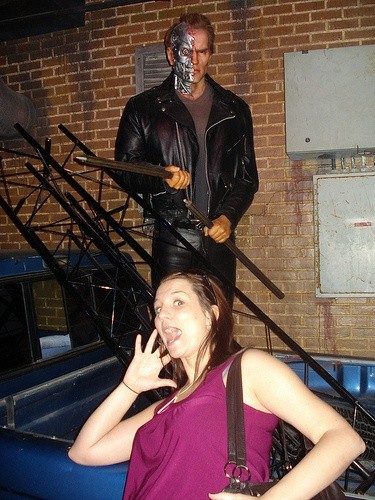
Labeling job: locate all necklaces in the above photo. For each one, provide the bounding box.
[157,377,198,415]
[121,380,140,395]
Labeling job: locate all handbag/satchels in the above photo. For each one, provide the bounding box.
[222,347,347,500]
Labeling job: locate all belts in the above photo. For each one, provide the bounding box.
[176,220,205,232]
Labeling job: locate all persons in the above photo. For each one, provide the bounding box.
[69,269,367,500]
[115,14,259,313]
